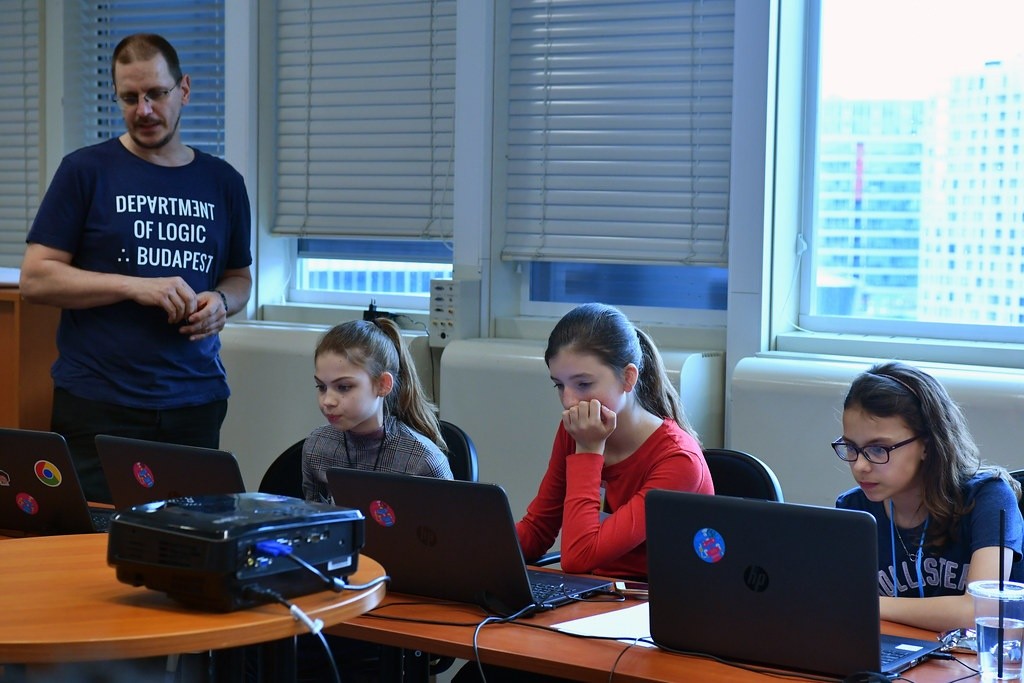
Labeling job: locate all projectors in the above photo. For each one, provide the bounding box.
[107,491,366,614]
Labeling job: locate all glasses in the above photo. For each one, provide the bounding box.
[112,79,180,111]
[830,435,921,465]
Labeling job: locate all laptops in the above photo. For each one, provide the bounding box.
[0,427,114,534]
[95,434,246,510]
[642,489,946,683]
[325,466,615,615]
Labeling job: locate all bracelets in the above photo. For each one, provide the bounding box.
[208,288,229,315]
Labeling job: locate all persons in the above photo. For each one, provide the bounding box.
[512,298,714,587]
[20,33,254,507]
[300,316,456,511]
[834,360,1023,636]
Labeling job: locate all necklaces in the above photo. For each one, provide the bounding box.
[889,509,924,562]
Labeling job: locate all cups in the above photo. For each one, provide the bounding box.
[965,580,1024,683]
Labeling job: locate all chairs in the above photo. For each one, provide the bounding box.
[533,448,784,565]
[258,420,478,682]
[1008,469,1024,585]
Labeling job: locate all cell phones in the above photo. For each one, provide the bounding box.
[615,581,648,596]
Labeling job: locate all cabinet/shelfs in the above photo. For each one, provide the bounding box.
[0,282,63,433]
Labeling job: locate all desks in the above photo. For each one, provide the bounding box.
[0,532,388,683]
[85,500,1024,683]
